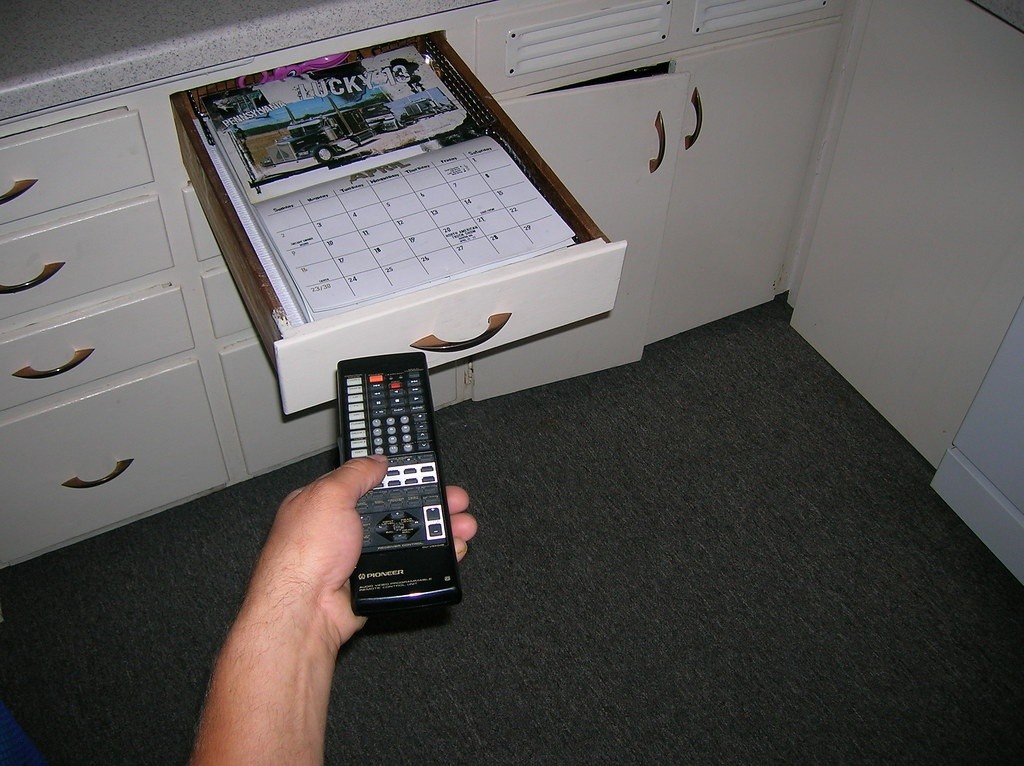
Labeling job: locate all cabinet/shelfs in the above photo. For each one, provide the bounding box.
[789,1,1023,469]
[473,1,843,405]
[170,33,626,474]
[930,303,1023,587]
[0,110,229,568]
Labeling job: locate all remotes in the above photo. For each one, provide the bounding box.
[334,353,464,617]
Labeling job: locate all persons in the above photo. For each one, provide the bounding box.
[185,454,478,766]
